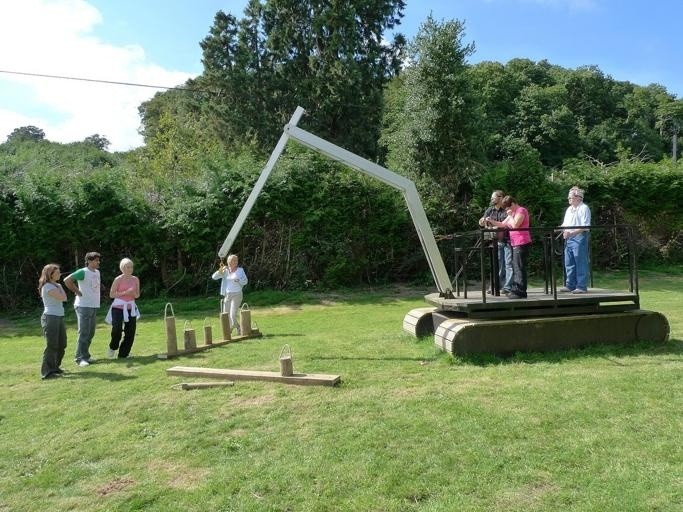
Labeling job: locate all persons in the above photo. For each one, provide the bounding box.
[108,257,141,360]
[63,251,101,366]
[486,195,532,299]
[37,264,67,379]
[212,254,248,334]
[479,190,513,294]
[559,186,591,294]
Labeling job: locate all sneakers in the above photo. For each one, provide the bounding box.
[501,290,521,299]
[78,357,95,366]
[237,326,240,334]
[572,289,586,294]
[108,350,116,358]
[561,287,572,292]
